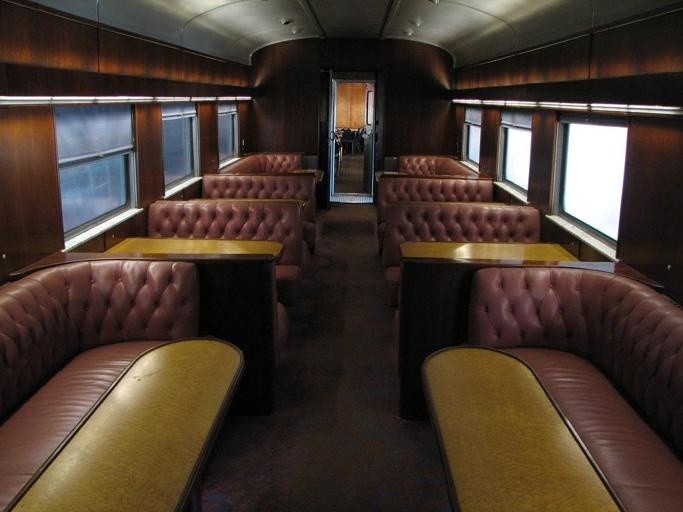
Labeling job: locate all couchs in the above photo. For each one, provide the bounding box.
[143,149,322,362]
[375,153,545,358]
[419,267,683,511]
[1,261,249,512]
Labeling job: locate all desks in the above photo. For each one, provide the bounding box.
[3,335,246,512]
[419,343,628,511]
[393,239,586,416]
[98,235,286,414]
